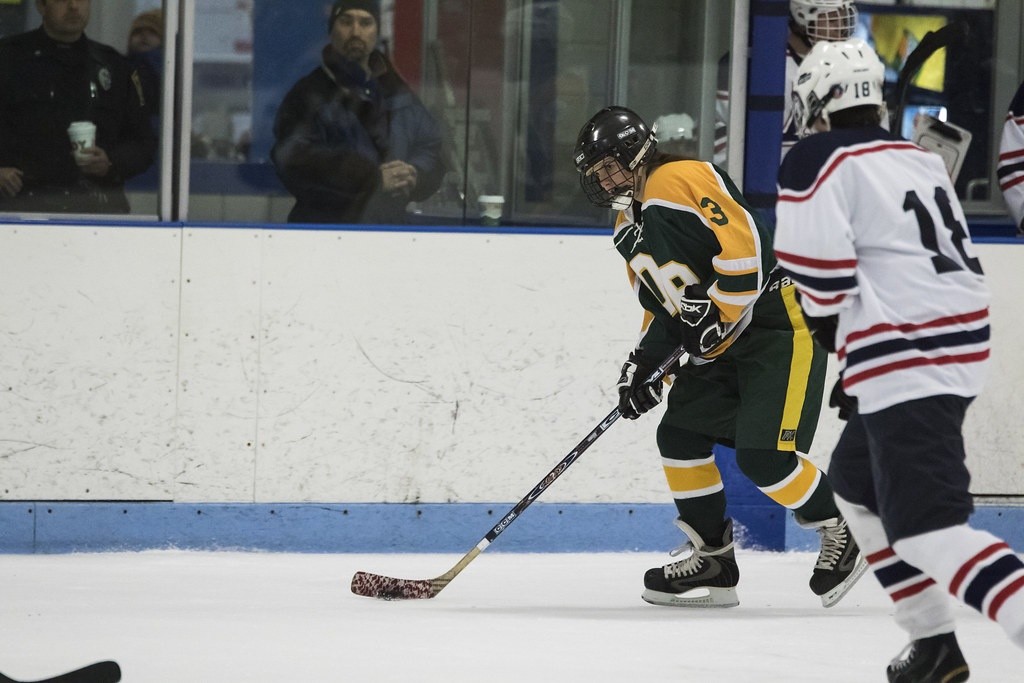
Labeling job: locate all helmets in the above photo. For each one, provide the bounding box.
[652,113,700,142]
[572,106,657,211]
[792,37,886,139]
[791,0,855,26]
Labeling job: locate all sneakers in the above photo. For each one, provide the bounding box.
[887,632,970,683]
[641,516,740,606]
[792,502,869,607]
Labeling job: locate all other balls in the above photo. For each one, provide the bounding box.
[378,590,401,600]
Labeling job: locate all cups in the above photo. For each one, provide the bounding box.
[476,196,506,227]
[68,122,96,164]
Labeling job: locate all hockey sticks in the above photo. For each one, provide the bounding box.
[1,661,122,683]
[351,343,687,600]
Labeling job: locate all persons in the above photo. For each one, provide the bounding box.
[713,0,889,172]
[773,38,1024,683]
[270,0,446,224]
[997,82,1024,233]
[127,6,209,161]
[0,0,158,214]
[573,106,860,594]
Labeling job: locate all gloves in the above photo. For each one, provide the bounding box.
[618,349,664,420]
[680,286,726,357]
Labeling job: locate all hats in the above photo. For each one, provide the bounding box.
[129,11,164,48]
[329,0,381,35]
